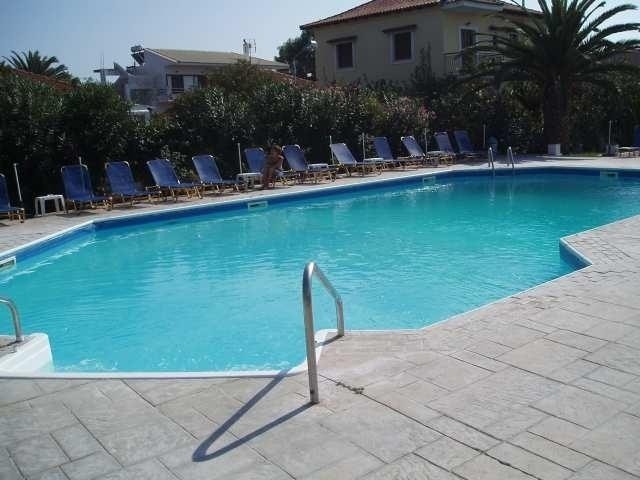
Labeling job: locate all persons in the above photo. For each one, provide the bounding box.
[260,144,284,190]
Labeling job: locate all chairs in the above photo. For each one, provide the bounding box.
[615,124,640,158]
